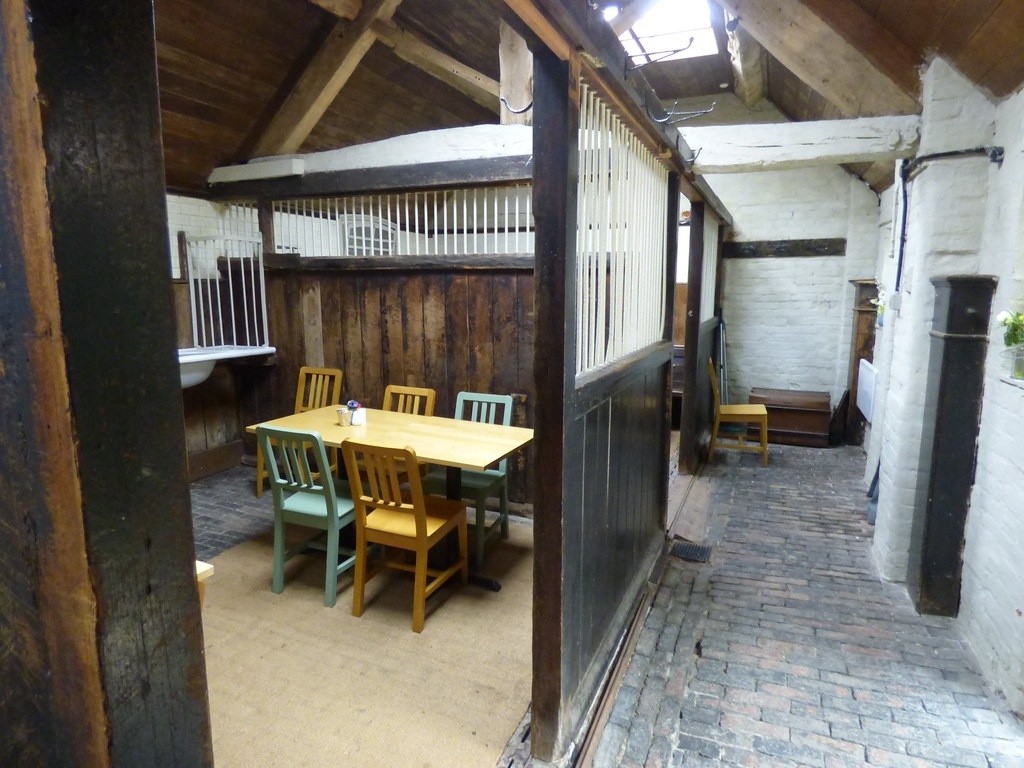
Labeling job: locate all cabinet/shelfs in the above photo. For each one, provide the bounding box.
[844,278,880,449]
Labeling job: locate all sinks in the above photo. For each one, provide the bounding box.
[178,348,217,388]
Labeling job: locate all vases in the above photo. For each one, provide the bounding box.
[1010,342,1024,380]
[877,312,883,327]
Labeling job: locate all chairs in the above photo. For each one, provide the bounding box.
[353,384,436,495]
[420,391,513,570]
[708,357,769,468]
[256,425,385,607]
[256,365,343,498]
[341,438,469,634]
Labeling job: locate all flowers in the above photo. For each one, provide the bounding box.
[868,291,885,305]
[995,308,1018,326]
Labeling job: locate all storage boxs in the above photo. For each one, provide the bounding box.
[746,387,830,449]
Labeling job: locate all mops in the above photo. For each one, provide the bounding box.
[719,319,746,432]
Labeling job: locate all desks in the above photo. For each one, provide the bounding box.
[246,403,534,592]
